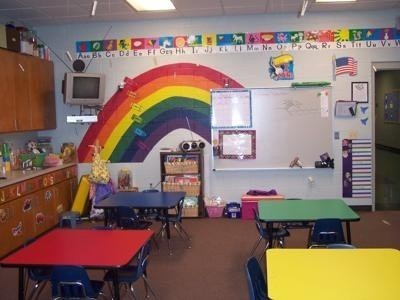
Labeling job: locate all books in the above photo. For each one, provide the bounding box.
[164,154,199,209]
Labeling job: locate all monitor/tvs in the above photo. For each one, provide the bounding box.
[60,72,105,107]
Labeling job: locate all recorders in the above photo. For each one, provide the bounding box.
[179,141,205,151]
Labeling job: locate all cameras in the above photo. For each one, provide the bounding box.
[315,157,334,168]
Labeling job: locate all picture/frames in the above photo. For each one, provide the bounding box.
[351,81,369,104]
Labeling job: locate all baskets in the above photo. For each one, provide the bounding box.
[164,160,199,174]
[168,207,198,217]
[163,181,201,197]
[204,202,226,218]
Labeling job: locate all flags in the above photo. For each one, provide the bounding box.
[334,56,359,76]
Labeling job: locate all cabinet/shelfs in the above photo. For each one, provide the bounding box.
[42,163,78,236]
[0,176,41,258]
[1,49,33,134]
[29,55,56,131]
[160,150,205,218]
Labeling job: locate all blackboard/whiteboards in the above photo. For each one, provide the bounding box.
[211,85,335,172]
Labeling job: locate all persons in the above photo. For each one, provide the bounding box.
[344,170,351,187]
